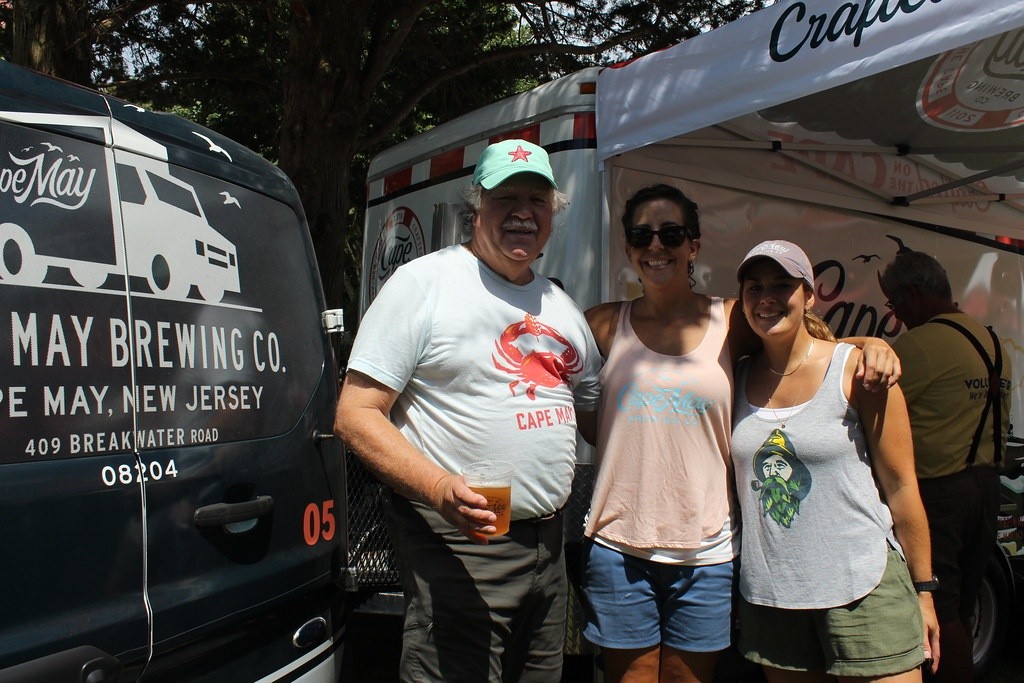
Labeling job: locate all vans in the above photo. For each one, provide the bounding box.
[0,54,353,683]
[347,67,1023,683]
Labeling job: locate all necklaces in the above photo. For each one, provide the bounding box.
[763,340,813,428]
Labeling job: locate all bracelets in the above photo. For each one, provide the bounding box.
[911,576,940,591]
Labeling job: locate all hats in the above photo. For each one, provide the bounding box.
[472,139,558,190]
[738,240,814,291]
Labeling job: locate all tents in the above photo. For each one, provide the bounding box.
[596,0,1024,298]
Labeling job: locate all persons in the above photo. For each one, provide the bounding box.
[878,251,1012,683]
[583,184,901,683]
[730,240,940,683]
[333,139,605,683]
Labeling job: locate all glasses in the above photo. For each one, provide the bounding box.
[628,224,690,248]
[885,298,897,311]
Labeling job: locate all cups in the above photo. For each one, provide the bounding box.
[461,461,513,537]
